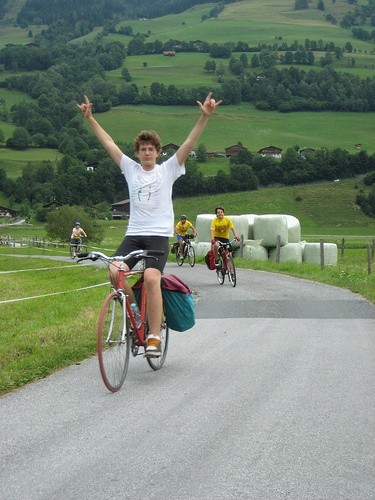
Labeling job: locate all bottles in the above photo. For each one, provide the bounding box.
[131,303,140,328]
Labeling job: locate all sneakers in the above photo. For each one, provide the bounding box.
[145,337,162,358]
[125,313,142,336]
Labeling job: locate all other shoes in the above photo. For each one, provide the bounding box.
[215,258,219,266]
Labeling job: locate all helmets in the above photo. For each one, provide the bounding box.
[180,215,187,220]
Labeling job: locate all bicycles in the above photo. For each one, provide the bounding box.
[70,236,87,260]
[215,239,238,288]
[175,234,197,268]
[76,250,170,394]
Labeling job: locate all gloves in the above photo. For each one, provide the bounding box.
[235,237,241,242]
[211,239,215,245]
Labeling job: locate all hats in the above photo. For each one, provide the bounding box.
[75,222,80,224]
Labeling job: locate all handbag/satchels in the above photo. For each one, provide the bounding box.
[127,274,142,308]
[204,249,216,270]
[171,243,179,254]
[161,274,196,332]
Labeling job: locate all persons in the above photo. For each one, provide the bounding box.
[71,221,87,257]
[210,207,240,280]
[77,92,223,355]
[174,215,196,259]
